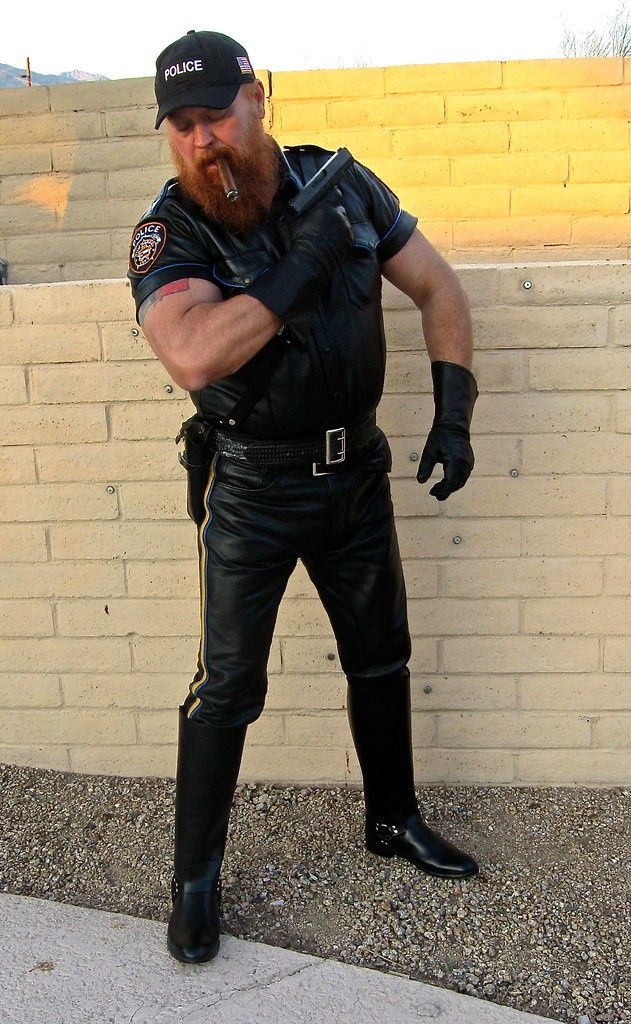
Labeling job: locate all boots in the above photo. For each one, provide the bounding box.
[167,705,248,964]
[347,666,479,879]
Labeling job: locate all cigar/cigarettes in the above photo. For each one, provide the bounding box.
[214,155,239,201]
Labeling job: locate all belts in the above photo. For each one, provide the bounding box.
[210,410,376,466]
[313,462,334,476]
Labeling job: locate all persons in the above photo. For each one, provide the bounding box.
[125,30,481,963]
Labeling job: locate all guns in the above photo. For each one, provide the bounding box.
[286,146,355,216]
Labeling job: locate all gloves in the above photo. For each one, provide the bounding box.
[239,185,355,351]
[417,361,479,501]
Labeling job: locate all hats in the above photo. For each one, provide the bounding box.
[155,30,256,130]
